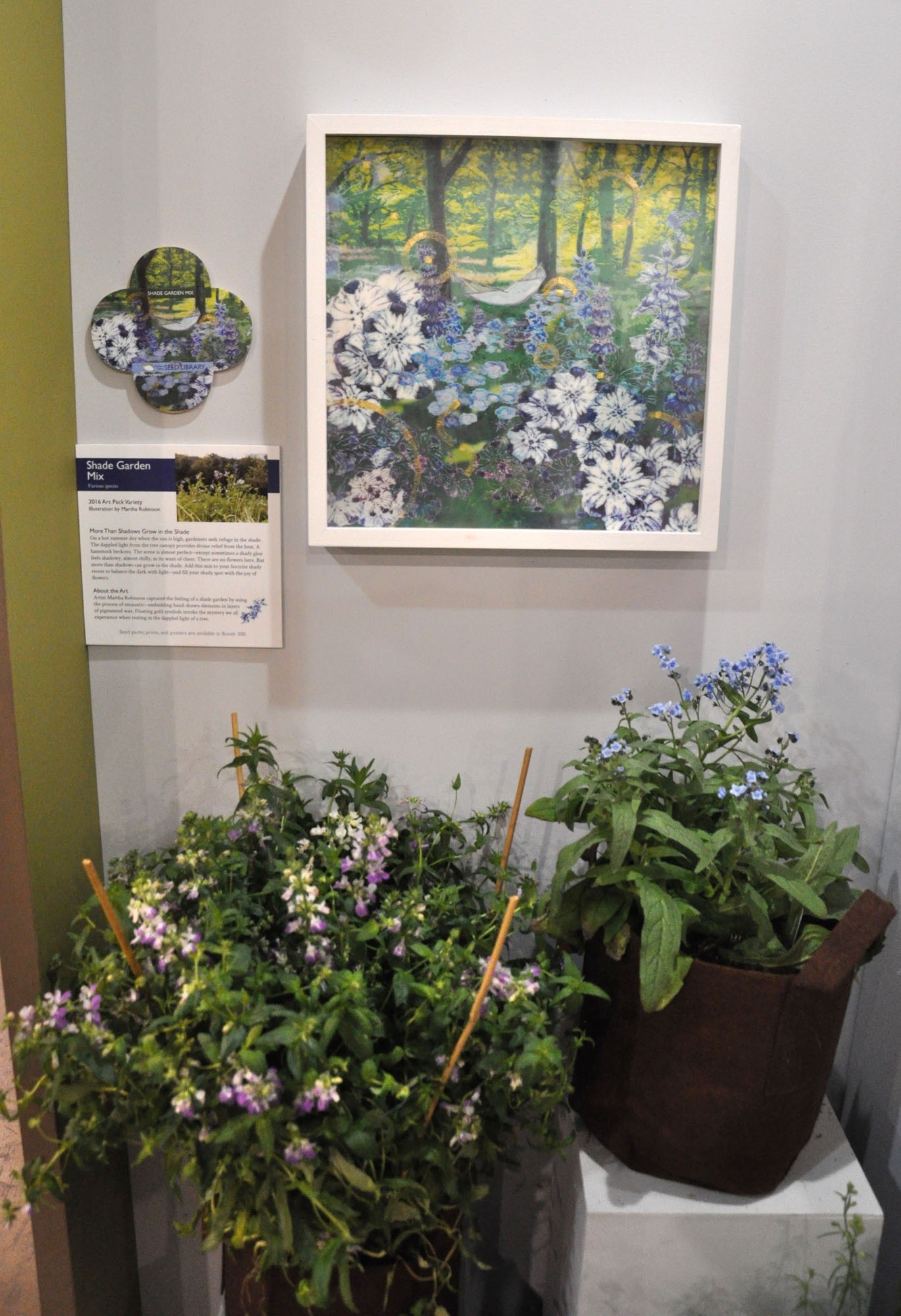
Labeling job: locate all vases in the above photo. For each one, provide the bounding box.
[218,1228,449,1315]
[557,884,901,1197]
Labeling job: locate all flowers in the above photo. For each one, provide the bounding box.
[516,629,878,1021]
[2,707,620,1316]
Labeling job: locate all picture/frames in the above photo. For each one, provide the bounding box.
[300,109,746,541]
